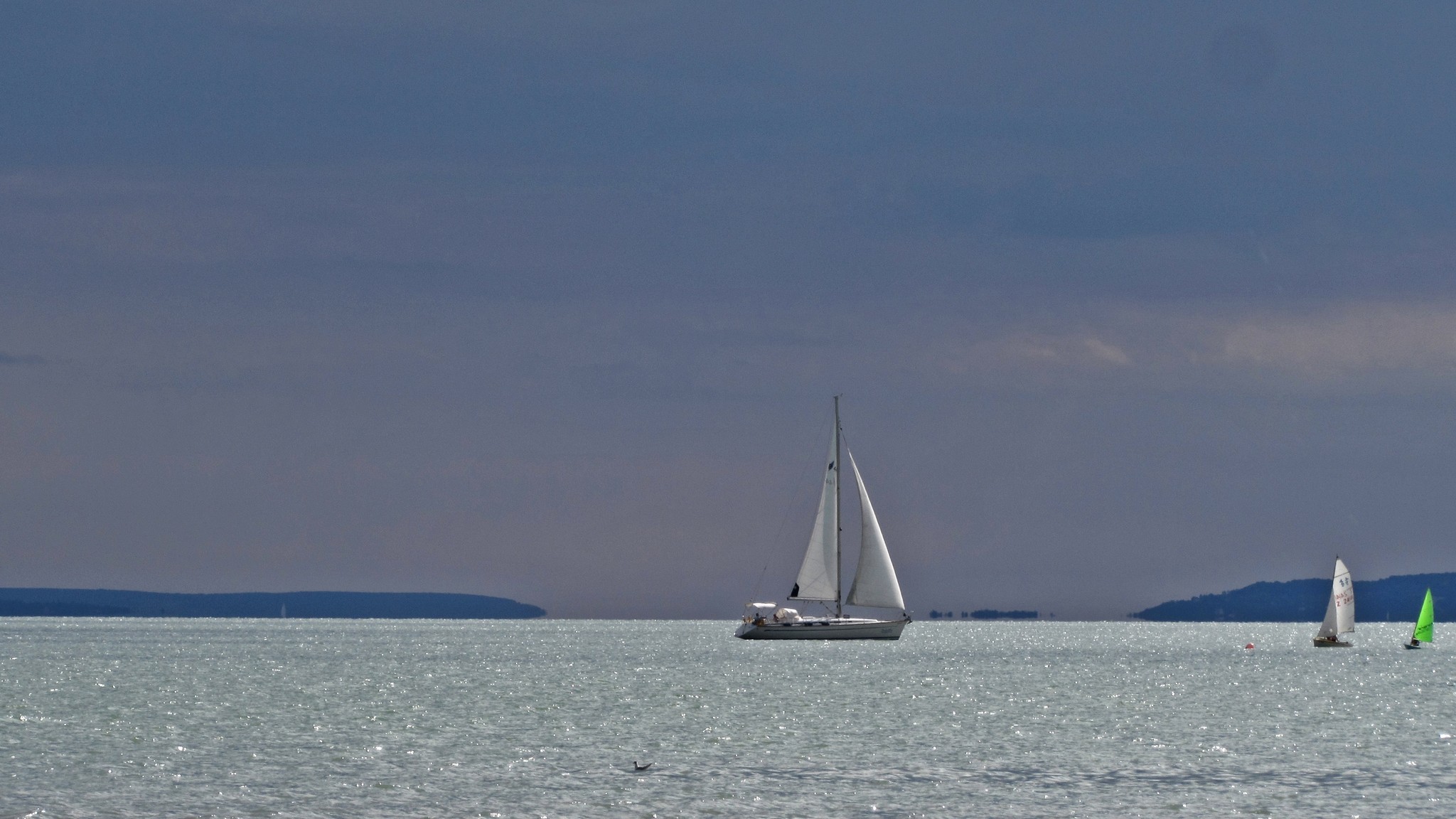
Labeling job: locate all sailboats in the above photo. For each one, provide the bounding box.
[1311,552,1356,647]
[733,395,912,641]
[1404,587,1434,651]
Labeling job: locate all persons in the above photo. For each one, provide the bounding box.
[773,614,780,623]
[752,613,767,626]
[1326,635,1339,642]
[1412,636,1421,646]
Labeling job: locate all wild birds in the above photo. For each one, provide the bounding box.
[632,761,652,770]
